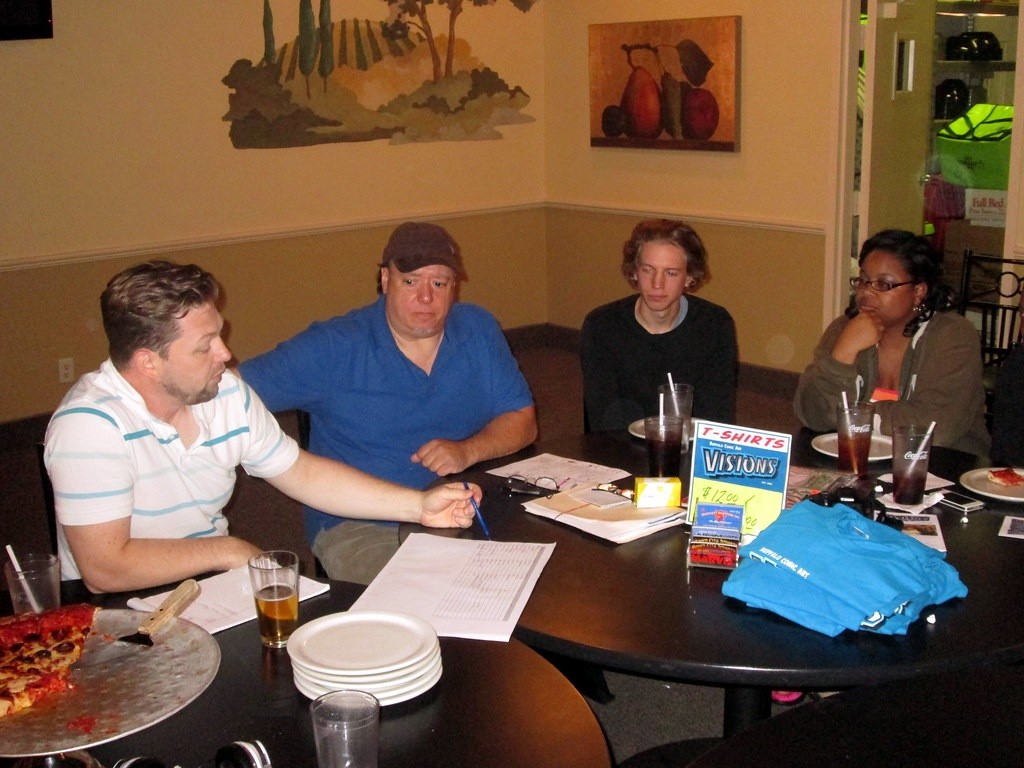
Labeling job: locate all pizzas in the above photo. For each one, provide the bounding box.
[987,468,1024,486]
[0,602,96,717]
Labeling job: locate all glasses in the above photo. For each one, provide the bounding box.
[849,277,920,292]
[497,474,560,499]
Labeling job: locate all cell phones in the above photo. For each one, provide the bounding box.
[930,490,984,512]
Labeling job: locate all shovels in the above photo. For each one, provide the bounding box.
[91,579,199,667]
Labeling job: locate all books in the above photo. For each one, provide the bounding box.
[127,557,330,634]
[521,485,685,543]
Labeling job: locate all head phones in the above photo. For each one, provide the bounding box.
[113,741,271,768]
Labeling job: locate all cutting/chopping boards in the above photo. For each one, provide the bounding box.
[0,609,221,757]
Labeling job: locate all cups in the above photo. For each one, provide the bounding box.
[644,415,683,478]
[5,553,61,616]
[310,689,380,768]
[836,401,876,480]
[658,383,694,438]
[892,426,935,505]
[248,551,301,648]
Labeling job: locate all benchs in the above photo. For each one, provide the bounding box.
[0,324,804,613]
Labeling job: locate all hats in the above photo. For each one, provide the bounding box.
[380,221,464,273]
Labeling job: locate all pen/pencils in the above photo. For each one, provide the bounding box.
[463,480,491,540]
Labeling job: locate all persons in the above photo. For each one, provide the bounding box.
[579,220,740,423]
[771,228,989,704]
[42,258,483,593]
[228,222,536,585]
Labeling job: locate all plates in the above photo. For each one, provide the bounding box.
[959,466,1024,503]
[287,611,443,707]
[628,416,704,440]
[812,433,893,462]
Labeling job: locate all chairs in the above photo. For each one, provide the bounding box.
[958,249,1024,439]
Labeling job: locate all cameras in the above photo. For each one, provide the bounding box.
[807,487,874,522]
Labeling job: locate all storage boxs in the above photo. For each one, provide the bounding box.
[946,221,1004,296]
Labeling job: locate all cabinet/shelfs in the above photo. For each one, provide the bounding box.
[934,0,1020,127]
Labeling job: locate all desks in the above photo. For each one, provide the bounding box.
[398,426,1024,768]
[0,577,611,768]
